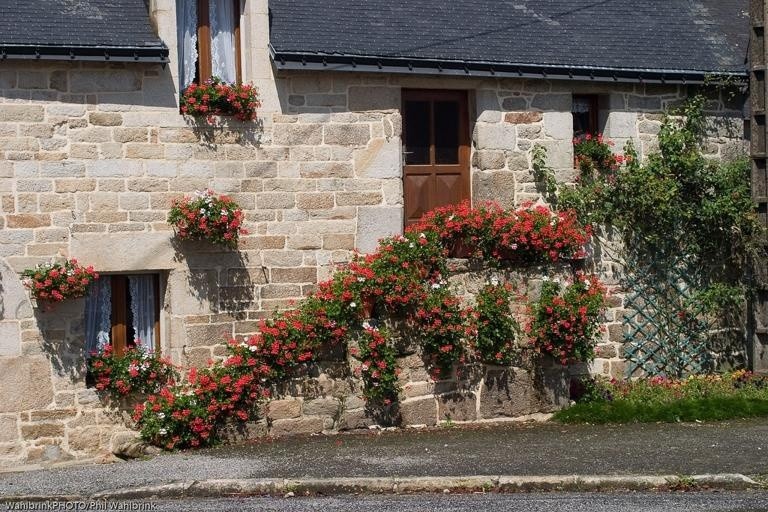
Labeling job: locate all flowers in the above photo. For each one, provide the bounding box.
[572,127,632,173]
[132,202,601,452]
[22,258,102,308]
[83,337,178,403]
[179,77,262,125]
[171,190,249,251]
